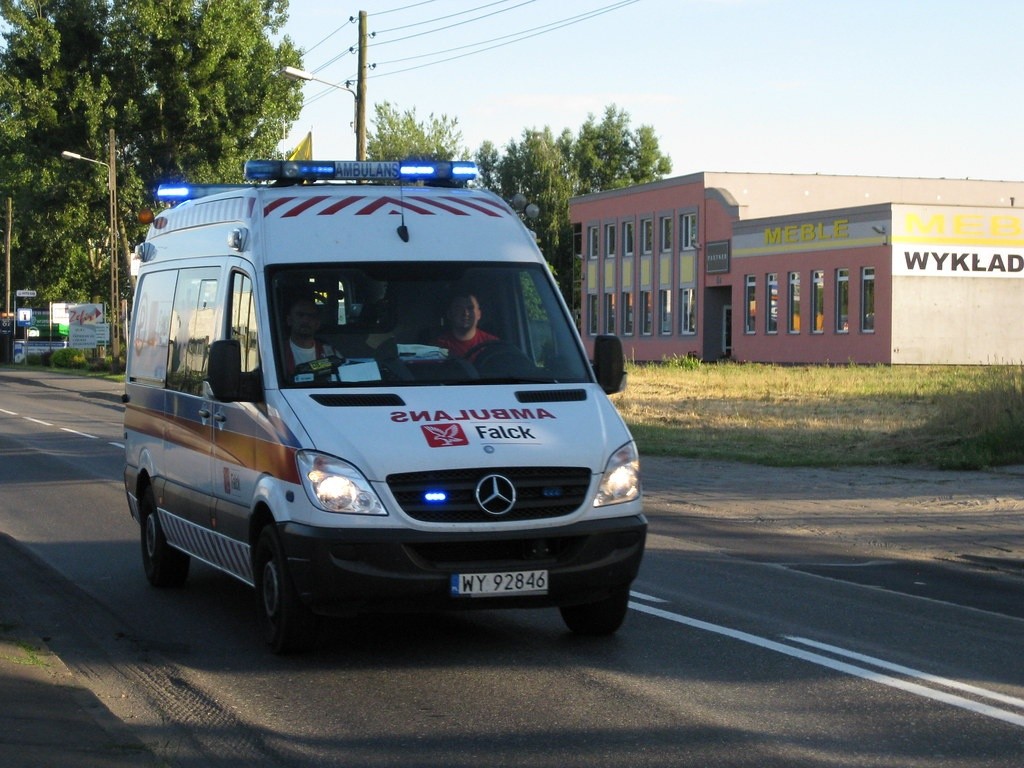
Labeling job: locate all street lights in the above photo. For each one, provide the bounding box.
[283,66,366,186]
[61,150,122,376]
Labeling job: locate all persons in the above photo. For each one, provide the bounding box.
[425,292,502,363]
[275,295,346,388]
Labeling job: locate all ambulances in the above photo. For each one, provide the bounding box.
[121,160,650,658]
